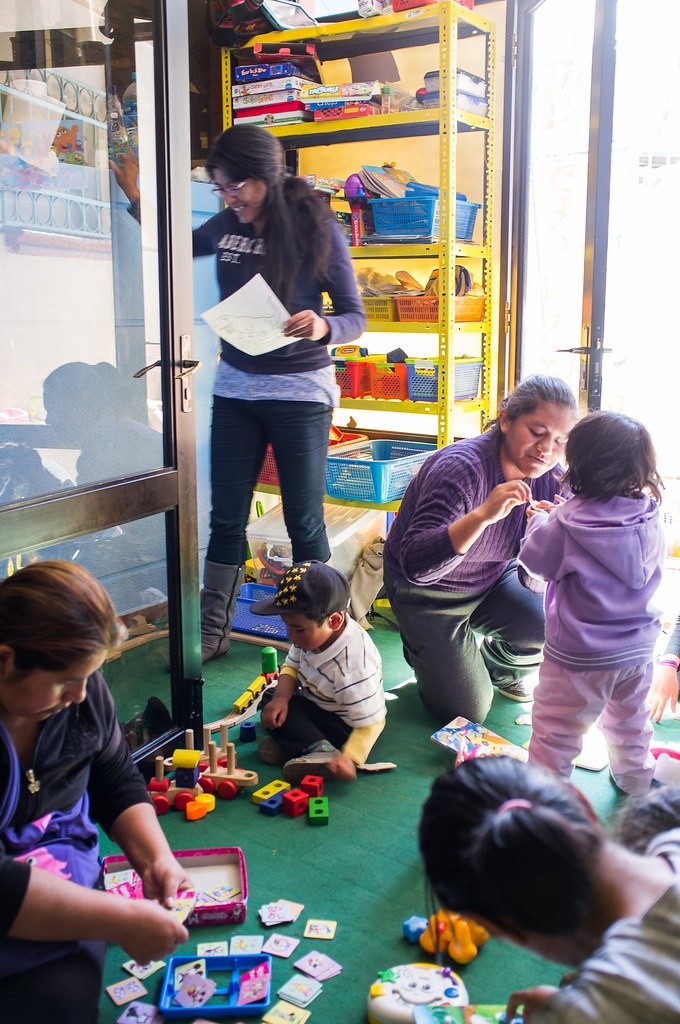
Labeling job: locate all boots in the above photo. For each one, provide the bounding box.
[199,557,245,664]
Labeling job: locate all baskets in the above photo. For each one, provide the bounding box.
[367,198,481,239]
[404,356,483,402]
[232,583,289,642]
[362,297,399,322]
[325,438,436,503]
[366,361,407,401]
[256,432,369,488]
[394,295,486,322]
[331,354,388,400]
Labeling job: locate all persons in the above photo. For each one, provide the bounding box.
[0,559,196,1024]
[648,615,680,722]
[417,754,680,1024]
[0,362,210,607]
[248,559,388,784]
[382,374,579,728]
[516,411,666,796]
[105,124,369,670]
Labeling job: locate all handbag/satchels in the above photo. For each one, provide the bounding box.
[0,814,101,977]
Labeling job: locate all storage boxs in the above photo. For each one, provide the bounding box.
[255,423,439,505]
[357,0,475,18]
[325,353,484,403]
[358,293,486,323]
[230,583,292,642]
[247,498,387,587]
[330,188,481,246]
[231,42,488,127]
[101,843,249,928]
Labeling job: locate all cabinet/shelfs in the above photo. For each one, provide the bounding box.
[220,0,504,610]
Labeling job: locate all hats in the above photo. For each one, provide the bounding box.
[249,560,351,615]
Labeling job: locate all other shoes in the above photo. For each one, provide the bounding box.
[283,742,340,782]
[259,736,287,764]
[496,681,533,702]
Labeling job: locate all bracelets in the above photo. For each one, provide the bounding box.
[659,659,676,666]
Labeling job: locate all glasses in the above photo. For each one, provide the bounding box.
[212,178,247,197]
